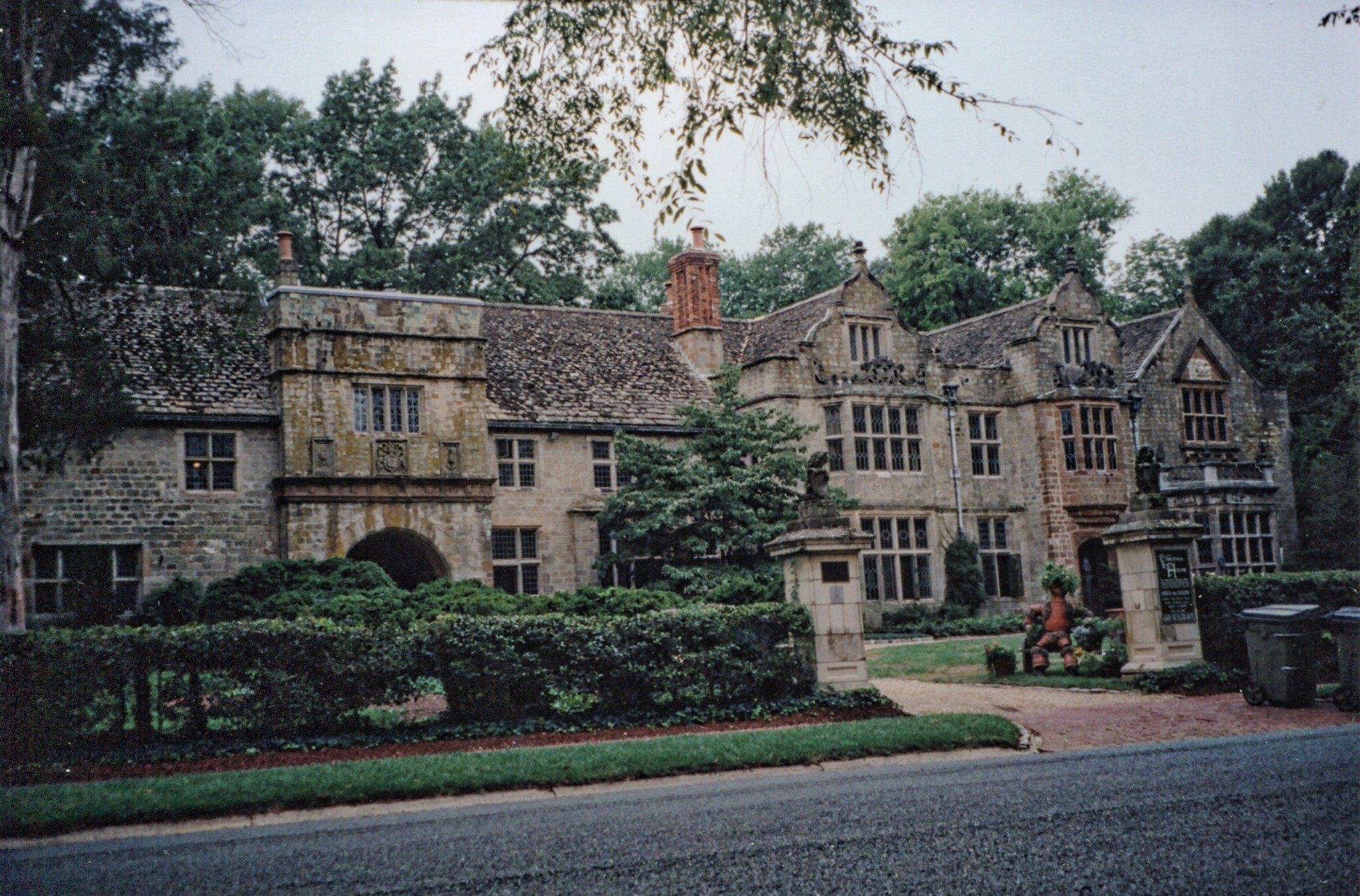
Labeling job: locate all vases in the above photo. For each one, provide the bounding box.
[1021,579,1079,671]
[993,654,1016,676]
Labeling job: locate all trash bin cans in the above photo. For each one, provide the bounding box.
[1233,604,1320,708]
[1325,607,1360,711]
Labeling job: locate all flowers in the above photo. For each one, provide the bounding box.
[979,638,1012,673]
[1040,562,1081,598]
